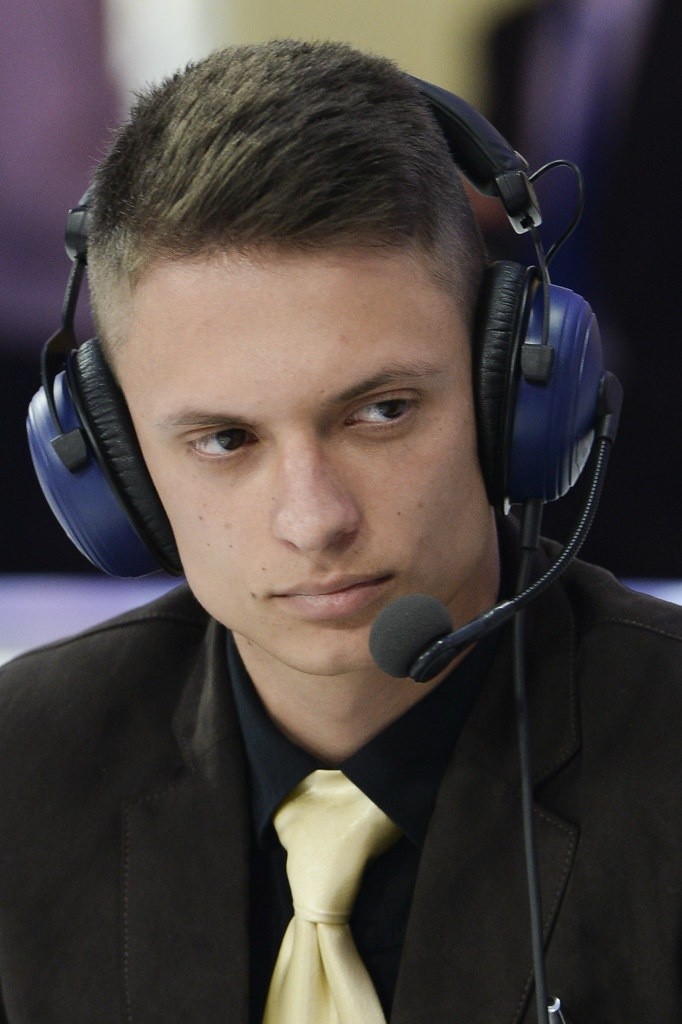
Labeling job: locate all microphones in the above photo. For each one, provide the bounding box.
[368,372,622,687]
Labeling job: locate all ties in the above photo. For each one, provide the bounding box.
[259,768,401,1024]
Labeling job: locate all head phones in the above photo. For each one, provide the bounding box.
[25,73,622,580]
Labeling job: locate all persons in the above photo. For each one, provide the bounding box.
[469,0,682,587]
[0,36,682,1024]
